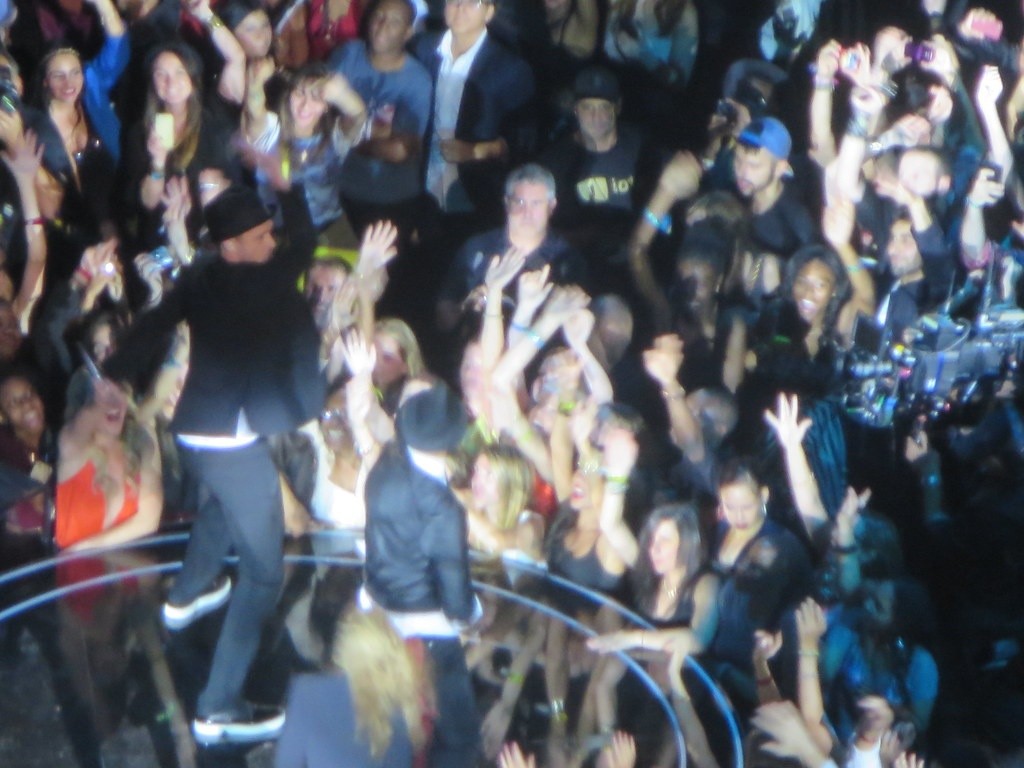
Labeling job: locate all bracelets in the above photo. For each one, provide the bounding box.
[661,388,686,400]
[148,171,160,178]
[814,82,831,89]
[482,312,504,319]
[76,265,92,283]
[353,439,372,453]
[606,475,628,484]
[644,208,672,234]
[967,197,984,208]
[527,330,544,349]
[24,216,43,225]
[509,320,526,330]
[207,15,224,29]
[606,485,629,493]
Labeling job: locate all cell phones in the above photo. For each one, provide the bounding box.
[155,113,175,151]
[980,161,1004,183]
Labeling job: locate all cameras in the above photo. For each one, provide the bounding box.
[149,244,173,267]
[903,43,933,61]
[838,51,859,70]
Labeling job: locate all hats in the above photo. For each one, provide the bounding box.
[200,186,278,239]
[742,118,792,176]
[395,387,468,451]
[574,65,620,104]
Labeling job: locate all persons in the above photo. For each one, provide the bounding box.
[0,0,1024,768]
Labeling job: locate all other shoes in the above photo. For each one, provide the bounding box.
[164,576,230,618]
[194,702,286,737]
